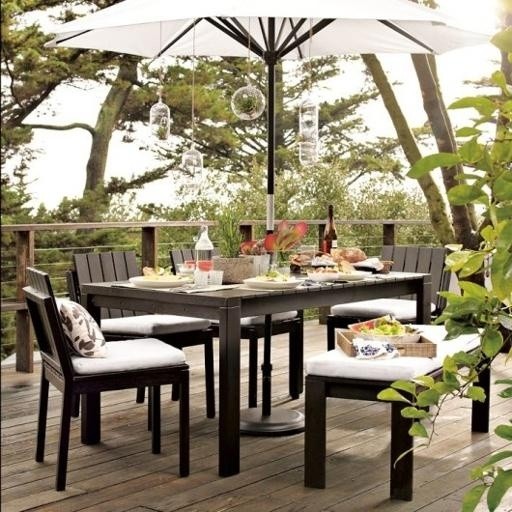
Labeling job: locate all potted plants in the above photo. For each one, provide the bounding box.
[212,206,254,283]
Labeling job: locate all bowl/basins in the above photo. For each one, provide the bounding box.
[308,268,339,283]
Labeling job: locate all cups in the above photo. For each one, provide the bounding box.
[194,272,207,288]
[175,263,185,277]
[185,261,195,275]
[207,269,223,284]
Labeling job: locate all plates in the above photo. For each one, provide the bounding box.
[340,271,372,280]
[244,278,305,288]
[129,276,191,288]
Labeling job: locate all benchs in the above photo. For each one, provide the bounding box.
[301,321,493,500]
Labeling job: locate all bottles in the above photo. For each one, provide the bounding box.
[322,205,338,253]
[193,225,215,272]
